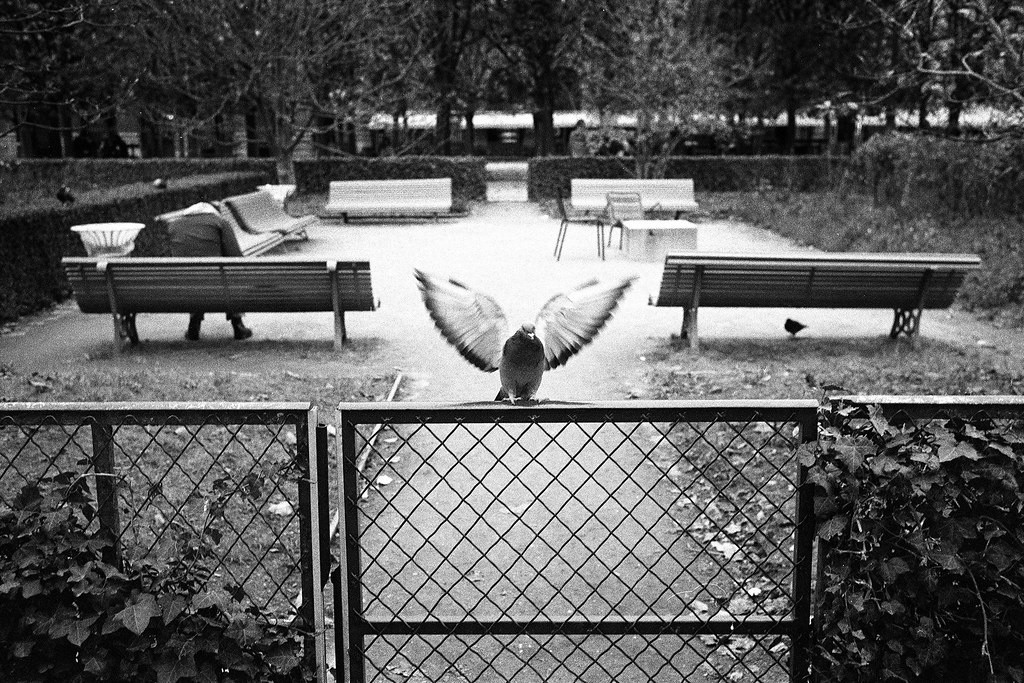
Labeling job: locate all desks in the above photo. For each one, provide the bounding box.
[620,220,698,261]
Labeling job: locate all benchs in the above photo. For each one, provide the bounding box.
[324,178,452,223]
[153,200,289,259]
[223,190,318,240]
[571,178,699,221]
[61,255,380,352]
[645,249,984,355]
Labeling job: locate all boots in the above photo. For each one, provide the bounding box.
[185,320,201,340]
[232,317,252,340]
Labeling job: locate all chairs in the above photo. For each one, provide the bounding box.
[553,185,608,260]
[605,191,665,250]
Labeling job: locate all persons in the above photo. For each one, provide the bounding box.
[164,202,253,340]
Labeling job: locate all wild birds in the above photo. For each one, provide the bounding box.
[412,266,641,407]
[783,318,808,338]
